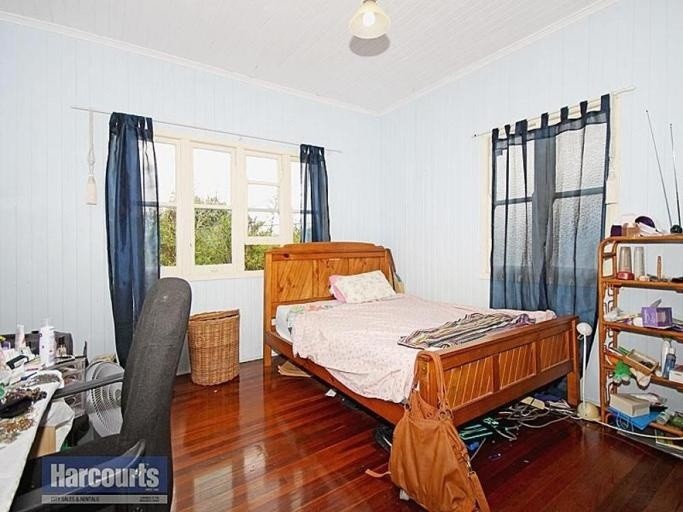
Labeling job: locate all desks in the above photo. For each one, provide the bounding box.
[0,340,65,511]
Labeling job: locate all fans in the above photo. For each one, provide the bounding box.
[80,358,125,439]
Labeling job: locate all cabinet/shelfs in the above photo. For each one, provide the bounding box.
[596,234,682,461]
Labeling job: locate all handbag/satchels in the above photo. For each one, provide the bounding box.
[389,350,491,512]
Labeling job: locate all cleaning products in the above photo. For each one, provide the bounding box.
[660,337,676,379]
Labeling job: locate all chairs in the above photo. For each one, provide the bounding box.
[13,277,191,511]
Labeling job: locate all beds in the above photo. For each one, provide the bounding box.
[258,240,587,503]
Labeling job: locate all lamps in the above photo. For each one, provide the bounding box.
[574,318,600,426]
[348,0,391,42]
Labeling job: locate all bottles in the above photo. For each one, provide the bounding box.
[633,245,645,280]
[14,324,25,351]
[38,318,55,367]
[661,348,676,378]
[661,339,670,372]
[619,246,631,275]
[56,337,66,358]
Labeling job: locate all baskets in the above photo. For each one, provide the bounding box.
[187,309,240,386]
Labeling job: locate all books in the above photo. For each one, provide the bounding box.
[655,429,683,451]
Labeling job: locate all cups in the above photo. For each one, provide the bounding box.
[656,411,669,425]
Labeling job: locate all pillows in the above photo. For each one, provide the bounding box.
[327,269,397,305]
